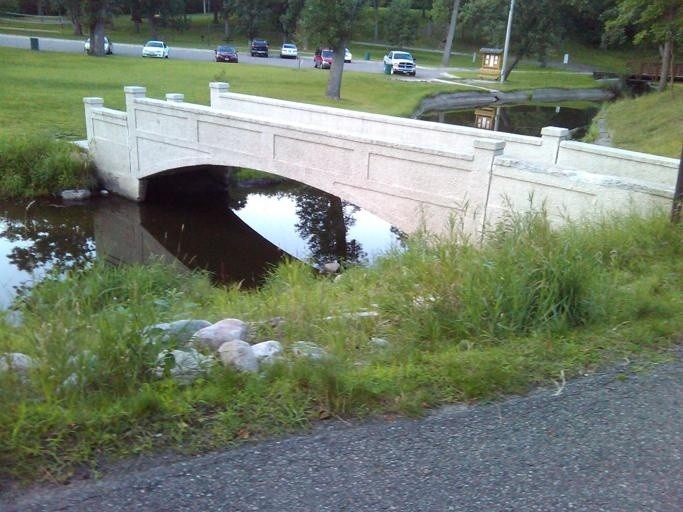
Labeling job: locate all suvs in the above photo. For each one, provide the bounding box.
[250,40,269,57]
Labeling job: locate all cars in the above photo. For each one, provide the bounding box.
[343,48,352,63]
[213,45,239,63]
[141,41,168,59]
[279,44,297,59]
[83,36,113,56]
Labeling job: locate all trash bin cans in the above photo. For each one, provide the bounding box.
[31,38,39,51]
[384,64,392,75]
[364,53,370,60]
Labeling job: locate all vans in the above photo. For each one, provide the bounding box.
[313,49,333,69]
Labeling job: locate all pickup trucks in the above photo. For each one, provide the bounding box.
[382,51,416,76]
